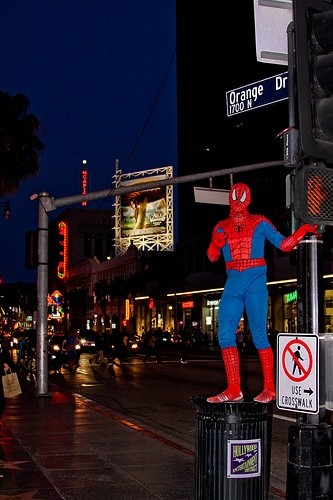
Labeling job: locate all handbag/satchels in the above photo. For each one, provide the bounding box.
[2,369,22,398]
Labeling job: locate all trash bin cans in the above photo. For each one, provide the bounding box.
[190,394,273,500]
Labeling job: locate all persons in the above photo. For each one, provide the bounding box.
[66,328,130,369]
[236,326,258,353]
[143,329,161,363]
[0,325,28,366]
[206,183,319,404]
[180,327,210,364]
[0,338,17,423]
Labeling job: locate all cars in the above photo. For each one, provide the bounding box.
[78,331,103,352]
[47,335,80,359]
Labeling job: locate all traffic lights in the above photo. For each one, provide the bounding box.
[293,0,333,161]
[295,165,333,226]
